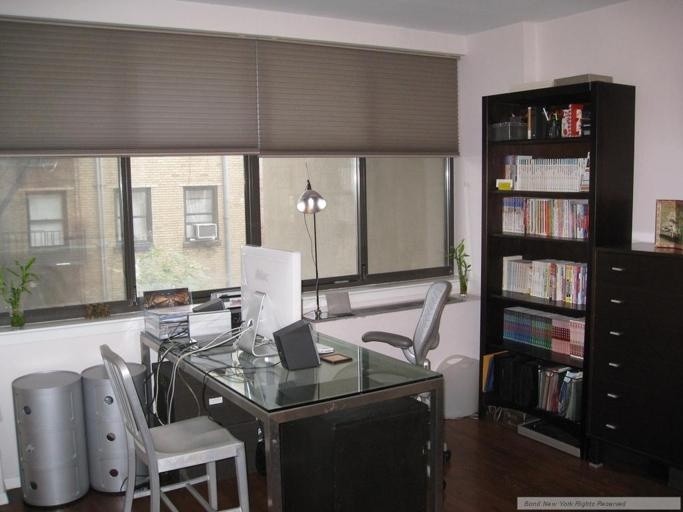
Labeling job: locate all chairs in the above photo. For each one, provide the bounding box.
[101,344,251,512]
[361,280,451,492]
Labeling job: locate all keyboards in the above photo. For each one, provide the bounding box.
[316,342,334,354]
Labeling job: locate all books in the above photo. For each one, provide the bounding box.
[502,255,587,305]
[482,306,586,422]
[502,197,590,239]
[514,152,590,193]
[526,102,584,138]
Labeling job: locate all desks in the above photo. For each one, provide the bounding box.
[140,332,444,512]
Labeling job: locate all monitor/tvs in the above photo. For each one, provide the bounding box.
[237,245,302,357]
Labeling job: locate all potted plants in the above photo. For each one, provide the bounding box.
[1,257,38,326]
[444,239,472,297]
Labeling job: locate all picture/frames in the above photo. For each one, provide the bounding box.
[655,199,683,251]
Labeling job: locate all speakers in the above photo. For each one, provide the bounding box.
[192,298,226,312]
[273,320,320,370]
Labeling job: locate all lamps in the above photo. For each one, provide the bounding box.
[295,178,341,324]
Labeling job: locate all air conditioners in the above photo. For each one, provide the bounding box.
[191,222,219,241]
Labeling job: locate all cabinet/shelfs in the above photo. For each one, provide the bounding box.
[584,240,683,491]
[479,81,636,464]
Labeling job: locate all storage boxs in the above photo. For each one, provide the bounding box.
[151,360,260,487]
[493,352,547,409]
[492,122,527,141]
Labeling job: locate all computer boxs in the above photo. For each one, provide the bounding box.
[152,360,258,485]
